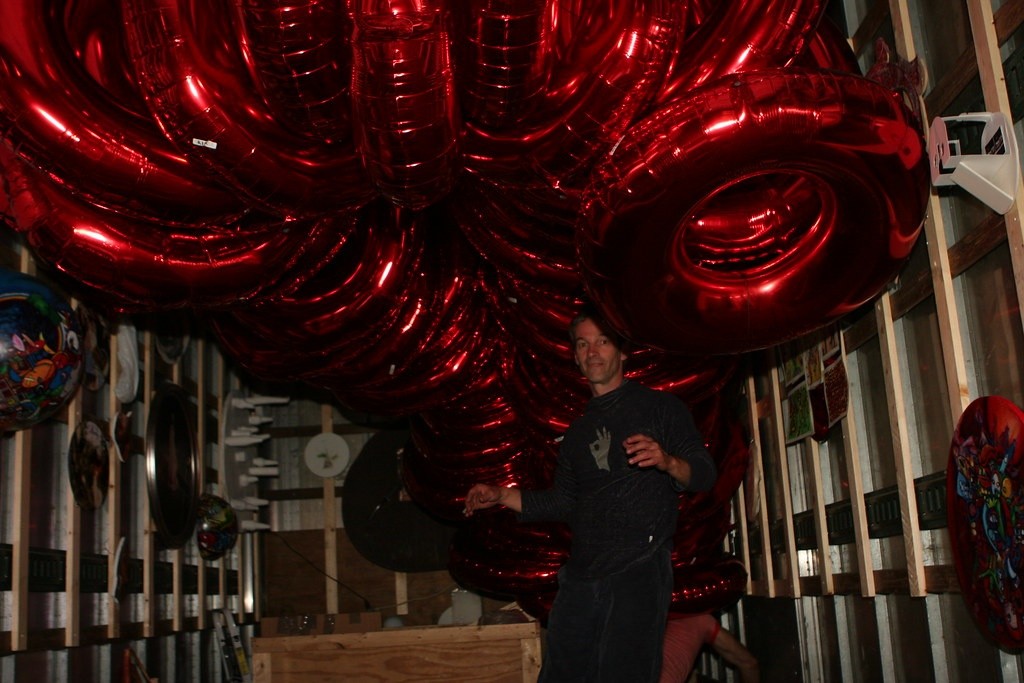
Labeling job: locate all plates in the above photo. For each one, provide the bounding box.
[69,421,110,510]
[305,432,350,478]
[0,272,84,432]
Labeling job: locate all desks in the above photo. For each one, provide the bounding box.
[248,622,544,683]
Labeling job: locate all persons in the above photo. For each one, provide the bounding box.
[465,311,718,683]
[659,614,760,683]
